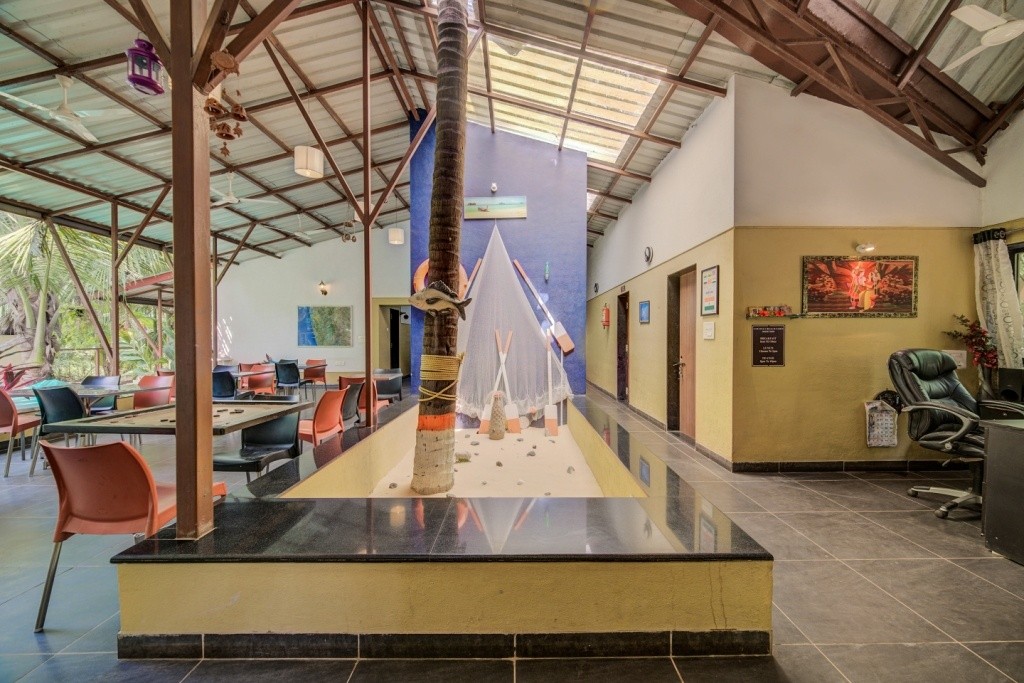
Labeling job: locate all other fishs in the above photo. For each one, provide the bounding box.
[408,280,473,321]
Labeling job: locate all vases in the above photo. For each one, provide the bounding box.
[979,345,997,395]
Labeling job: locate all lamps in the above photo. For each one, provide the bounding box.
[294,87,324,179]
[856,243,875,253]
[388,194,404,244]
[318,280,328,296]
[354,201,375,222]
[124,32,165,96]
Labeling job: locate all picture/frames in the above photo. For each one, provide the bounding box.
[700,265,720,316]
[639,300,650,324]
[802,255,918,318]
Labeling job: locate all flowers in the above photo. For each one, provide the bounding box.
[942,314,999,370]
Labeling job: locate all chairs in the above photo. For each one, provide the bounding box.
[0,359,403,633]
[887,348,1024,519]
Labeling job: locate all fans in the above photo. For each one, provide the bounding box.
[210,172,278,207]
[259,214,343,240]
[0,74,135,142]
[937,0,1024,73]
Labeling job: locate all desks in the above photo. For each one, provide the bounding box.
[978,419,1024,565]
[230,371,277,389]
[5,386,171,470]
[336,373,403,380]
[297,364,327,369]
[42,400,316,543]
